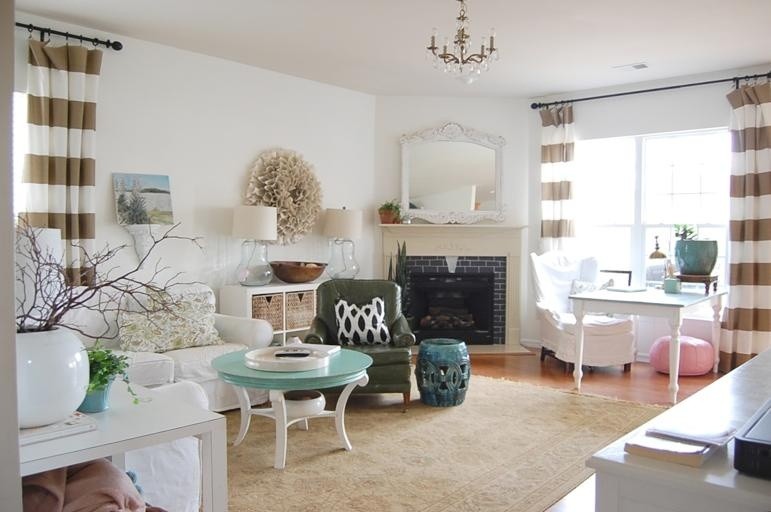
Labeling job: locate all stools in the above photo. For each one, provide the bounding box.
[417,338,471,407]
[650,336,716,375]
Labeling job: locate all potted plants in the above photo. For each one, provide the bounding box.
[78,349,145,413]
[671,222,720,275]
[380,203,402,224]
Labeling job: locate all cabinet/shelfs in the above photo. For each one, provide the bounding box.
[219,282,319,346]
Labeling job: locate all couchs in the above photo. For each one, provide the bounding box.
[77,284,273,411]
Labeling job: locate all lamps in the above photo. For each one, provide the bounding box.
[649,235,672,291]
[426,1,501,84]
[233,204,279,284]
[324,206,362,280]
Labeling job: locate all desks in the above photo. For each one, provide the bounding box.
[18,382,229,511]
[573,287,724,402]
[582,349,769,510]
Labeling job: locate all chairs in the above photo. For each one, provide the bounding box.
[526,252,632,373]
[305,280,415,407]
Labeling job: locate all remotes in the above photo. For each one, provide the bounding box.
[275,350,310,357]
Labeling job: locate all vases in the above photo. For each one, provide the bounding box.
[17,324,95,430]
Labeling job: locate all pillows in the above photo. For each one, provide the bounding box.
[120,285,222,351]
[330,297,394,347]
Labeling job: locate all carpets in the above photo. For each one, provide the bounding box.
[220,375,668,512]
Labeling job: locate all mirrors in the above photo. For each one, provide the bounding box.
[399,123,508,224]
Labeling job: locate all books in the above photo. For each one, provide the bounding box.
[624,414,738,470]
